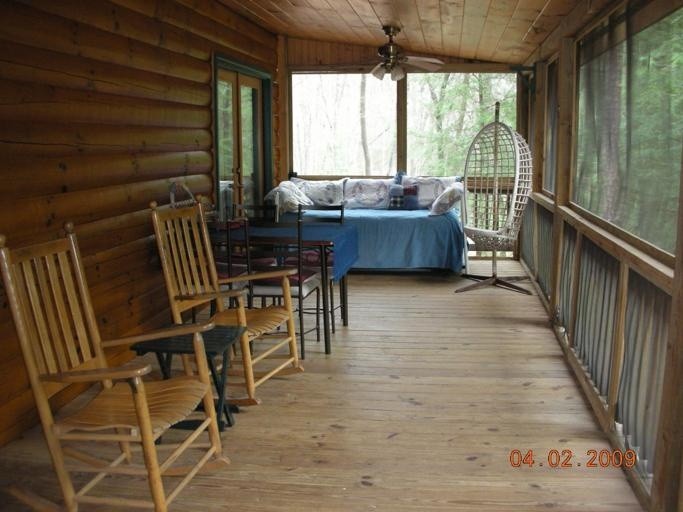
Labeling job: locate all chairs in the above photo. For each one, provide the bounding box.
[187,219,251,331]
[1,221,228,512]
[244,205,320,359]
[231,203,283,306]
[150,201,306,407]
[297,205,346,333]
[456,102,536,296]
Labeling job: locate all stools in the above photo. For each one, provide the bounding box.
[132,323,247,445]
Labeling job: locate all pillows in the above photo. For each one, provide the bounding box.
[402,176,458,208]
[430,183,463,215]
[267,180,313,212]
[290,178,347,208]
[343,177,393,209]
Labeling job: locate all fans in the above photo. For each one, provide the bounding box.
[342,26,444,73]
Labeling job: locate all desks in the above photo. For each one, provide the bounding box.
[148,222,356,355]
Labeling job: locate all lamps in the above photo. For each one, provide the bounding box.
[370,62,405,82]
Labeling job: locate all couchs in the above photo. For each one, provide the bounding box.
[273,178,463,276]
